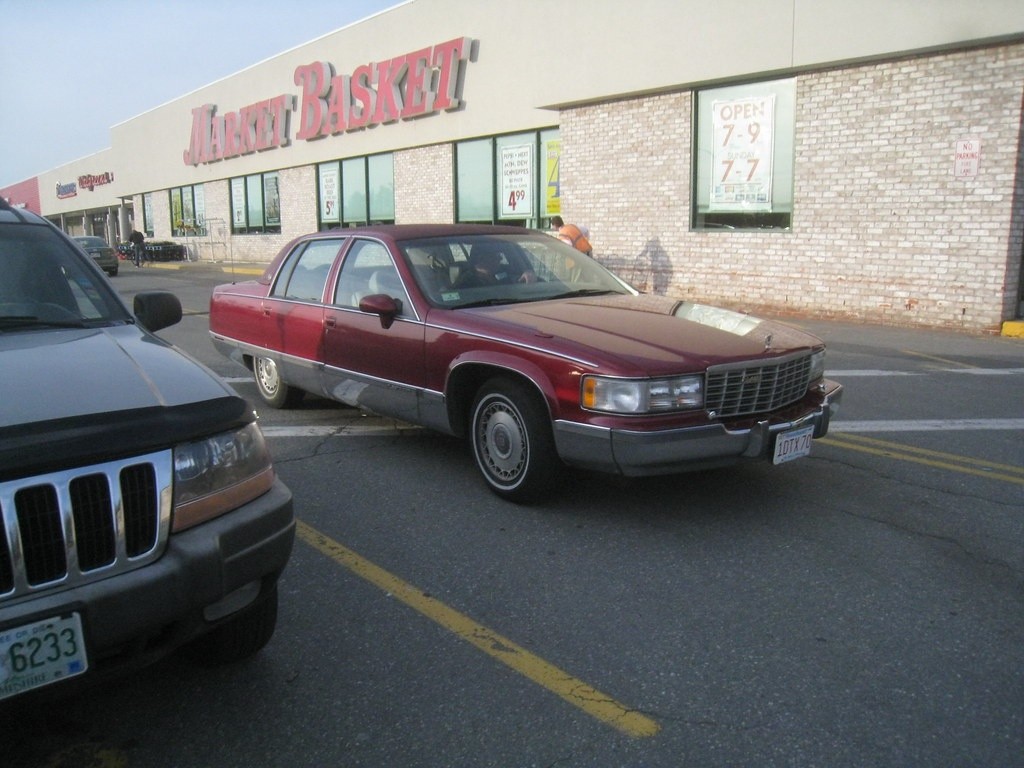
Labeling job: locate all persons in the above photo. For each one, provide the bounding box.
[129,230,149,268]
[194,225,204,235]
[551,216,593,282]
[177,225,182,236]
[185,225,195,236]
[454,242,535,290]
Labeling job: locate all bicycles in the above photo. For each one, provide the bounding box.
[131,245,145,267]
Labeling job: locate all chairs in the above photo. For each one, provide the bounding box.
[368,270,413,315]
[448,262,477,287]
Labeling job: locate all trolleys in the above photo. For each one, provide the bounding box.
[520,245,559,284]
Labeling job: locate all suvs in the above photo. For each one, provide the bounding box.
[0,196,298,714]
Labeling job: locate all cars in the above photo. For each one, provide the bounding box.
[206,220,841,500]
[68,236,119,276]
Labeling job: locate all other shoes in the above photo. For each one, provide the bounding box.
[136,266,138,268]
